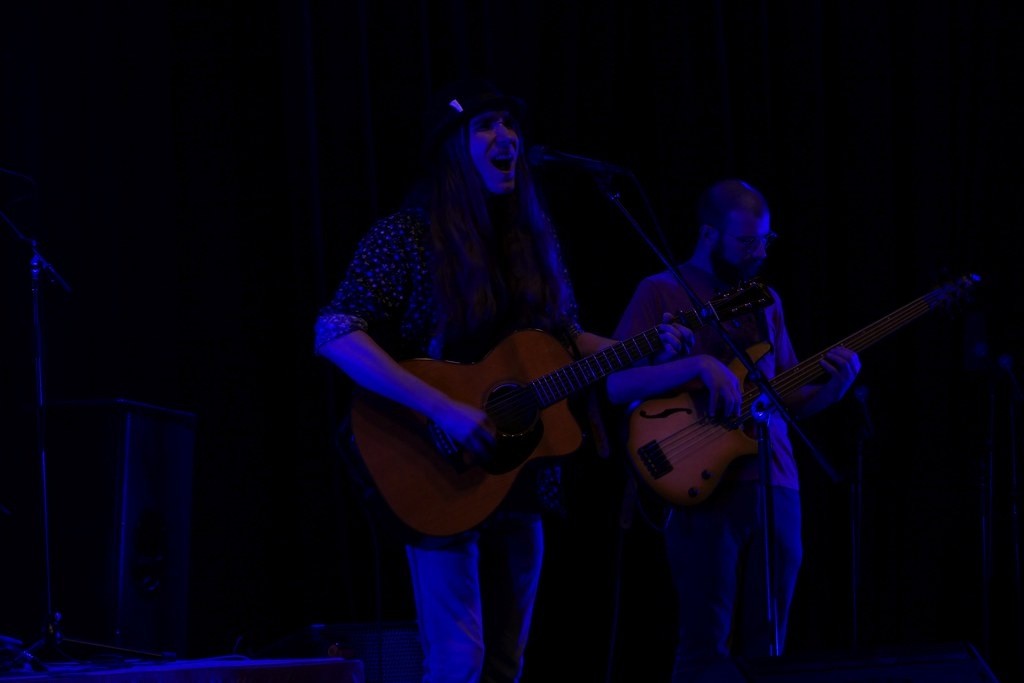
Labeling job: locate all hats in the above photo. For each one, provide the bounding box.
[418,75,527,162]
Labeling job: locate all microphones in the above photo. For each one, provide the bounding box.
[529,146,617,175]
[855,386,873,432]
[1000,355,1024,397]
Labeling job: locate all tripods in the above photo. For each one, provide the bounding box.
[0,212,178,672]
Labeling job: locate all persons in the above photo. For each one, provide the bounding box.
[606,179,862,683]
[312,76,693,683]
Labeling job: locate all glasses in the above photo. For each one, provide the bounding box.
[725,228,777,255]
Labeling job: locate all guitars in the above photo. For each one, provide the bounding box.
[346,278,778,550]
[619,263,992,510]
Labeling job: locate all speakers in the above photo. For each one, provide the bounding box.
[696,639,1001,683]
[41,396,198,660]
